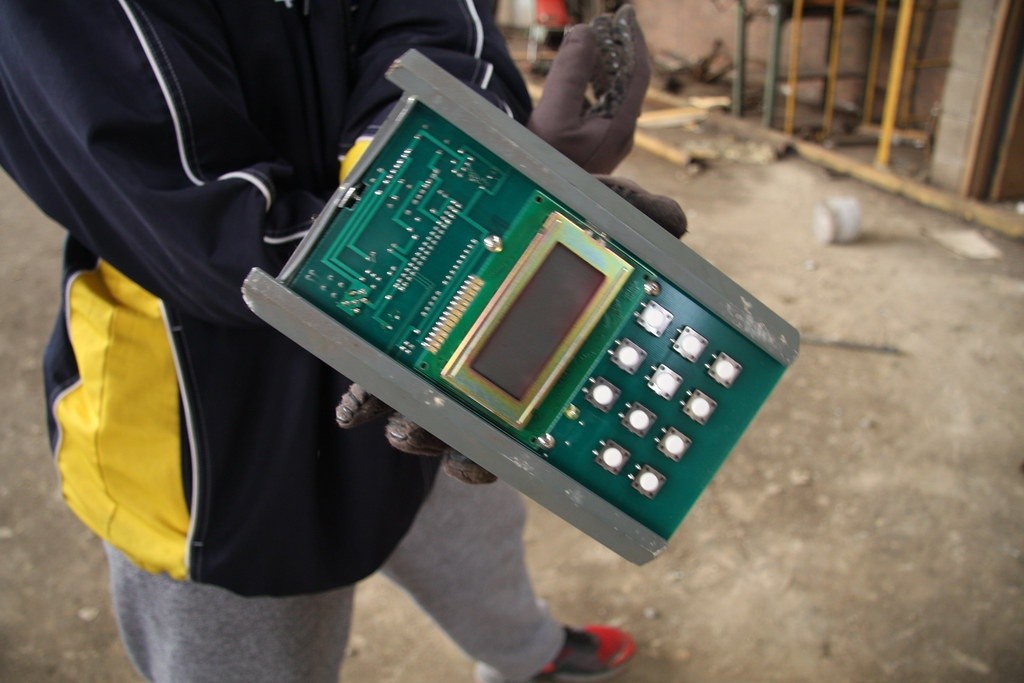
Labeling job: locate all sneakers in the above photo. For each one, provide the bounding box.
[531,619,640,682]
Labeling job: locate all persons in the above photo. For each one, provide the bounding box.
[0,0,690,683]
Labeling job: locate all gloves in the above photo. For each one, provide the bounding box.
[332,175,688,485]
[524,2,655,174]
